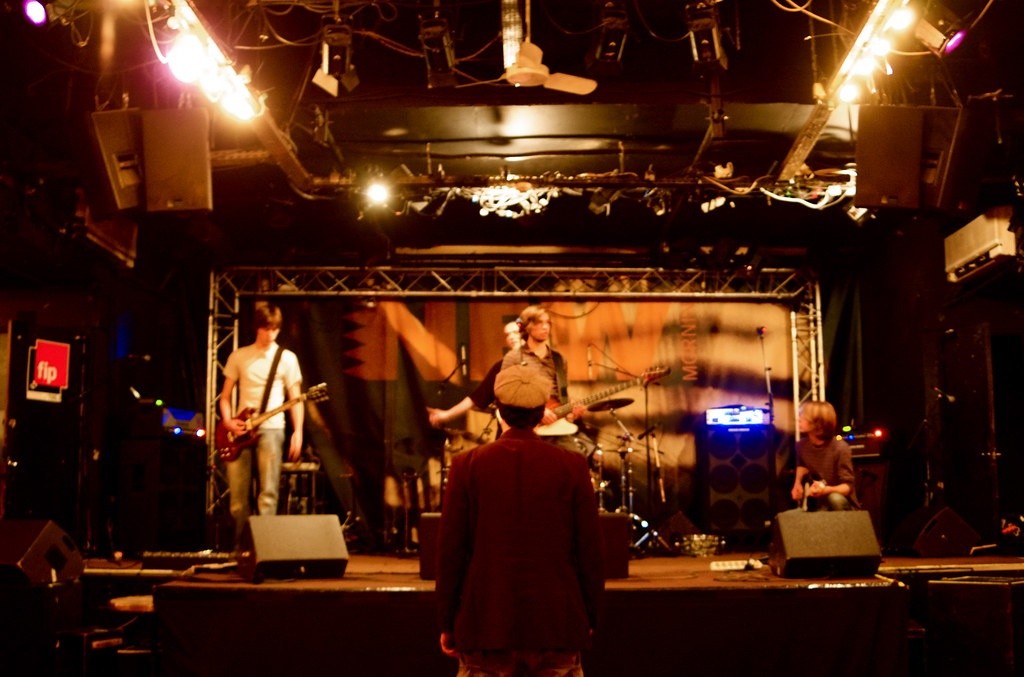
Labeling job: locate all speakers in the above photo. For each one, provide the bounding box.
[598,512,630,579]
[888,504,982,560]
[86,107,146,218]
[853,103,924,209]
[0,519,86,596]
[116,439,205,562]
[636,502,703,557]
[238,514,349,583]
[696,424,778,554]
[419,513,442,581]
[916,105,995,210]
[143,108,213,215]
[769,511,881,578]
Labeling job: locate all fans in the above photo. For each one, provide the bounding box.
[453,0,599,98]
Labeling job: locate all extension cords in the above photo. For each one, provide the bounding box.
[710,559,763,571]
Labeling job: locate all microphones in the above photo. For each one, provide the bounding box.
[461,345,467,376]
[637,425,657,439]
[588,345,593,378]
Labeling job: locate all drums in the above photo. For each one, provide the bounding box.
[628,512,670,558]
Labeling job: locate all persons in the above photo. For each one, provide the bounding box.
[790,401,861,513]
[220,306,305,558]
[424,304,589,438]
[432,363,602,677]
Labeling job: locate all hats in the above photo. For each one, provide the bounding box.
[494,361,552,409]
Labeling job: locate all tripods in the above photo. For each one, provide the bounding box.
[610,407,672,558]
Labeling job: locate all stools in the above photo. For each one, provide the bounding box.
[281,462,320,514]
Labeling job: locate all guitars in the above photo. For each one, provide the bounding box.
[495,363,673,436]
[215,381,330,463]
[797,478,826,511]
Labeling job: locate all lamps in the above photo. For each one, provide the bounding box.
[416,16,463,93]
[831,0,918,106]
[24,0,83,27]
[310,25,360,97]
[680,0,728,65]
[581,0,635,79]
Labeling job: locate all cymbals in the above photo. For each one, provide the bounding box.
[586,398,634,412]
[602,445,642,453]
